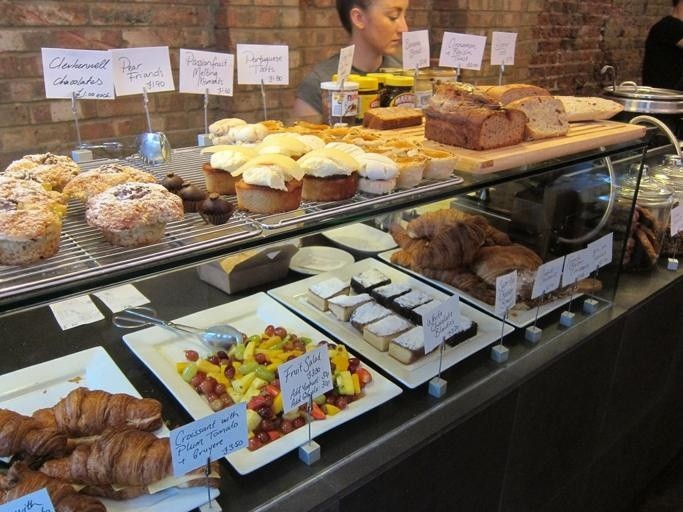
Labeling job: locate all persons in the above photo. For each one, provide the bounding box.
[291,0,408,126]
[642,0,682,90]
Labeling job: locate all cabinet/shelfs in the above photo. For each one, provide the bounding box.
[0,113,682,512]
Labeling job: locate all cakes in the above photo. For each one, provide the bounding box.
[61,163,158,204]
[0,172,69,267]
[420,80,625,153]
[84,182,187,249]
[160,118,459,226]
[306,266,479,366]
[360,104,423,130]
[5,151,81,192]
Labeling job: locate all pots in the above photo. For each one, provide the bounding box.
[602,84,683,146]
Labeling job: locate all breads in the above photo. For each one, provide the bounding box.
[391,212,604,314]
[0,386,221,512]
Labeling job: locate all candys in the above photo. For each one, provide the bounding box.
[175,323,372,453]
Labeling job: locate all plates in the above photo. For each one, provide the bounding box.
[0,344,222,512]
[264,254,515,393]
[118,288,404,480]
[321,223,398,254]
[285,245,354,276]
[379,246,586,329]
